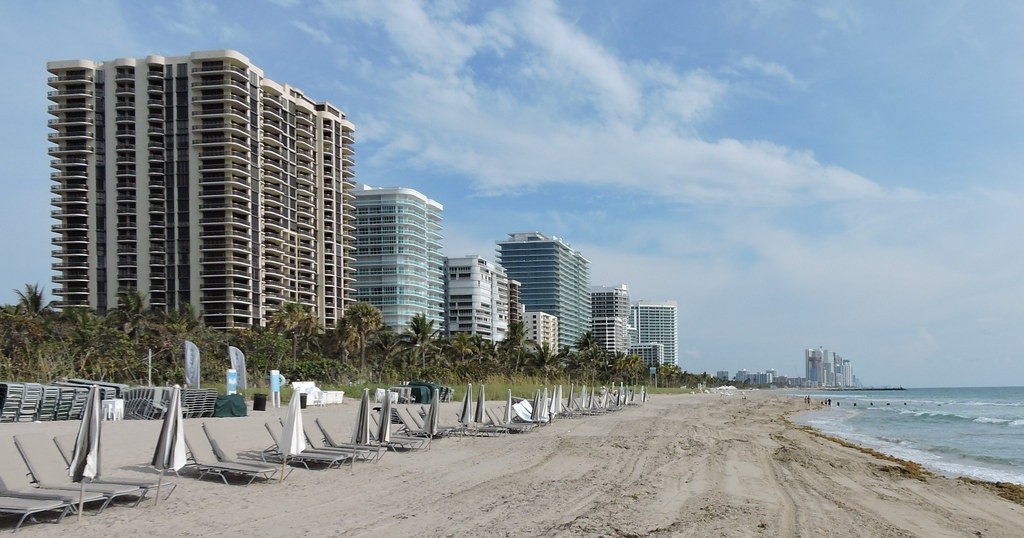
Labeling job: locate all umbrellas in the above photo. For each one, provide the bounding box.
[423,388,439,450]
[717,385,737,398]
[67,384,101,521]
[458,383,473,440]
[473,385,486,438]
[152,384,187,506]
[278,386,306,484]
[374,388,392,464]
[530,381,647,429]
[503,388,512,437]
[349,388,370,475]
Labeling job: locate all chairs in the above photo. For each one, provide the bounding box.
[374,381,454,403]
[0,403,622,535]
[291,381,345,406]
[0,379,219,423]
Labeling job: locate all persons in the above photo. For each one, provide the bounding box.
[804,395,810,403]
[824,398,831,405]
